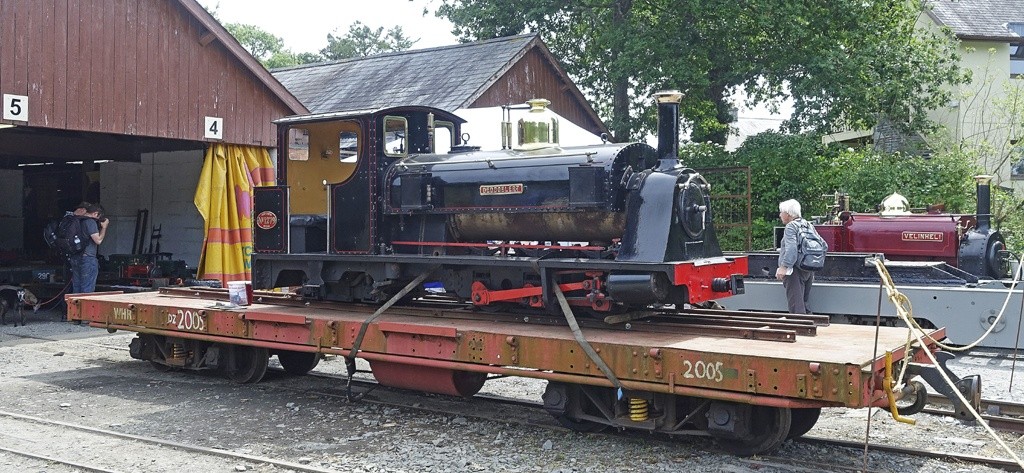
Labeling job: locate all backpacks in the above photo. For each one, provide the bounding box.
[43,220,58,247]
[793,221,827,272]
[55,217,98,253]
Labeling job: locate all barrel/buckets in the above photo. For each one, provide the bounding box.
[225,280,252,307]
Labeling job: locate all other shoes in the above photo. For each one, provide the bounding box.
[74,320,81,325]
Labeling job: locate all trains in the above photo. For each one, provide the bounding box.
[249,87,751,336]
[714,174,1023,289]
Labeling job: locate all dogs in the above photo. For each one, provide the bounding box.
[0,288,26,327]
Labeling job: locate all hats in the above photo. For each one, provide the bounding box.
[75,201,91,208]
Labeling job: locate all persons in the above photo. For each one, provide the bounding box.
[61,201,109,326]
[775,199,829,315]
